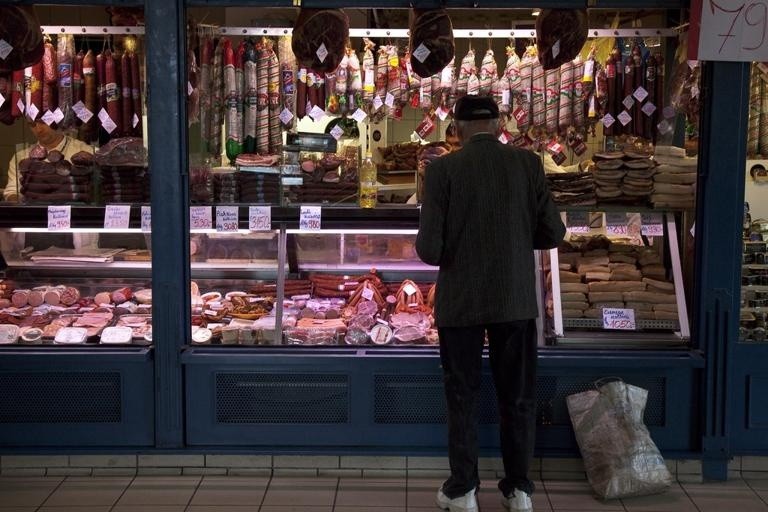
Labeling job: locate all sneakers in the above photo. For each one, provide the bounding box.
[436,483,480,511]
[500,490,535,511]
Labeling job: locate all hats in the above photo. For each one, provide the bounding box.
[448,94,502,121]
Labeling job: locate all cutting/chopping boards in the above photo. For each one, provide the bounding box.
[123,252,151,261]
[31,248,124,263]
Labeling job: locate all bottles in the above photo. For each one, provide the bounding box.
[360,152,377,208]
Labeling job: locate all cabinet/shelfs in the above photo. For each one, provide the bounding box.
[0,202,156,455]
[180,202,703,459]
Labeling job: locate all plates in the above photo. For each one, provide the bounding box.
[110,314,153,340]
[227,313,268,319]
[41,314,116,339]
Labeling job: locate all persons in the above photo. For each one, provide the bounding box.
[2,113,99,202]
[416,93,566,512]
[407,120,461,204]
